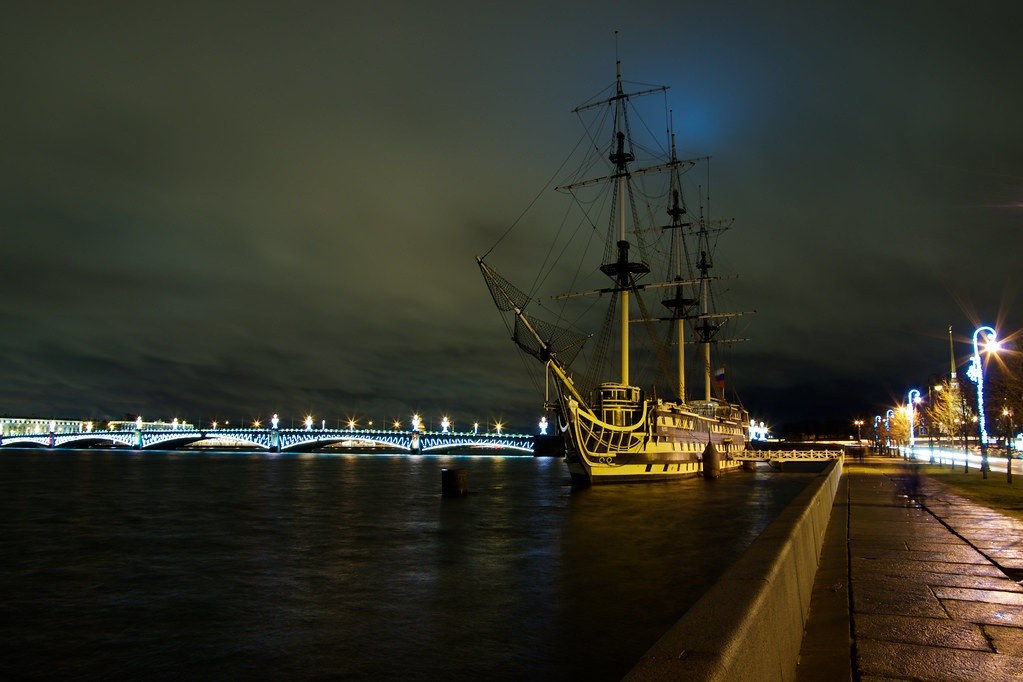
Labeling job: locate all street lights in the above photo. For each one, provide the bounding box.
[411,414,420,431]
[875,416,882,443]
[539,416,548,435]
[909,389,921,459]
[135,416,143,429]
[966,326,999,472]
[305,416,312,430]
[172,418,178,430]
[440,417,450,433]
[886,409,895,447]
[271,414,280,430]
[854,420,863,441]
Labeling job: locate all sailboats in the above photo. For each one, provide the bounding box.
[474,29,751,487]
